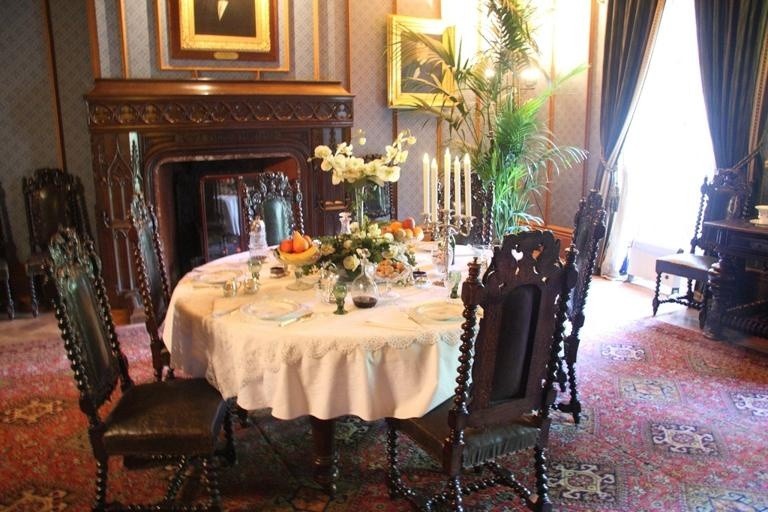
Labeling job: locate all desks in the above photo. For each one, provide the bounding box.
[696,215,768,344]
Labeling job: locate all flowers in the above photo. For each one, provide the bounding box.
[301,223,412,285]
[305,123,418,224]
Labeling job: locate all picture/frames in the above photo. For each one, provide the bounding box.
[385,14,462,111]
[165,0,282,66]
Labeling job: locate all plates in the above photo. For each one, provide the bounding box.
[749,219,768,229]
[197,269,241,283]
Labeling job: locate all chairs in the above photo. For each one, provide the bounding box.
[438,170,495,246]
[550,188,608,426]
[345,152,399,229]
[43,222,238,511]
[201,173,305,265]
[384,228,564,512]
[22,168,90,318]
[126,190,248,428]
[646,163,755,331]
[1,187,22,321]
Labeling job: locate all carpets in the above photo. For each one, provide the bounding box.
[1,265,768,509]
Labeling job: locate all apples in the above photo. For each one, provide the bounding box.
[280,240,293,253]
[382,217,423,243]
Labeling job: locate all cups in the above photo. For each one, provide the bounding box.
[754,202,768,219]
[223,278,240,296]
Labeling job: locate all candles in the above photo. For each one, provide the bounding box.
[453,154,462,217]
[443,148,452,210]
[429,157,438,223]
[463,152,472,217]
[422,152,430,216]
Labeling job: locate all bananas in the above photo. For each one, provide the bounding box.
[277,246,317,262]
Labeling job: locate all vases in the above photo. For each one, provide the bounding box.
[349,275,378,309]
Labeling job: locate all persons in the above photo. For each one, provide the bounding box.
[193,0,256,36]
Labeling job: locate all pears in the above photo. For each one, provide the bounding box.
[293,231,312,253]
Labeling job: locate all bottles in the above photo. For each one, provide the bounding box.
[241,211,468,313]
